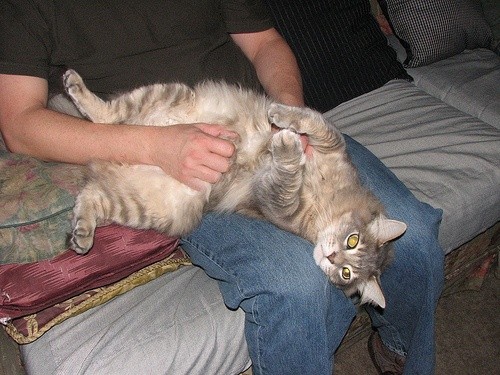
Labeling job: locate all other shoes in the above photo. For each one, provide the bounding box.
[368,329,407,374]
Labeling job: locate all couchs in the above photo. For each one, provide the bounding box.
[0,0,500,375]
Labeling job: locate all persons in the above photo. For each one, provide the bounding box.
[0,0,444,375]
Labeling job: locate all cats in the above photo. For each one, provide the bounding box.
[62,69,408,309]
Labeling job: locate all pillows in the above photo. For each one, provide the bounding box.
[384,0,497,70]
